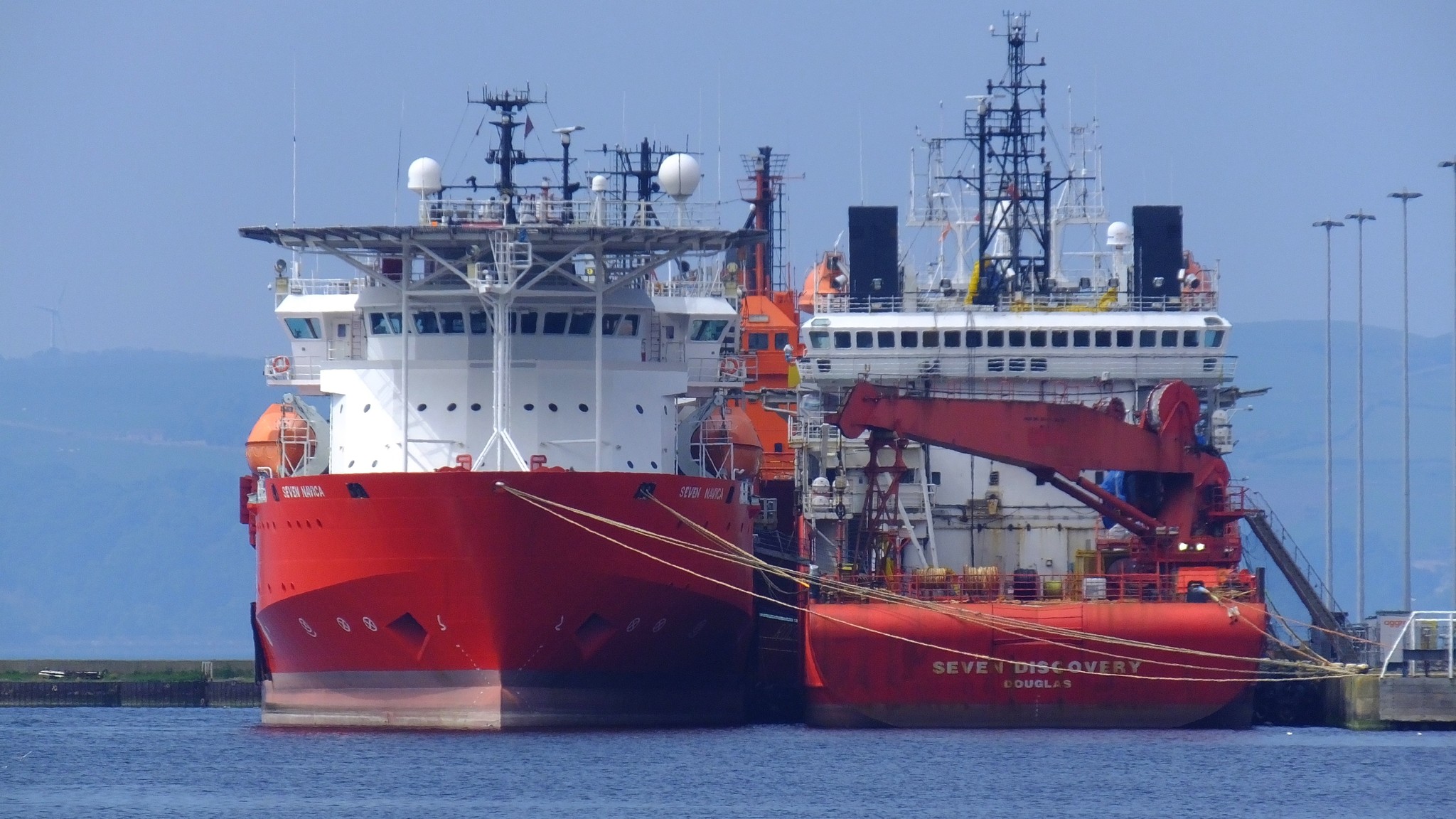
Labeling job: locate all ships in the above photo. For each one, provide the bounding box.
[237,8,1265,732]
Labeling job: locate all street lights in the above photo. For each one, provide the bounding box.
[1387,188,1426,613]
[1345,207,1377,624]
[1307,217,1346,613]
